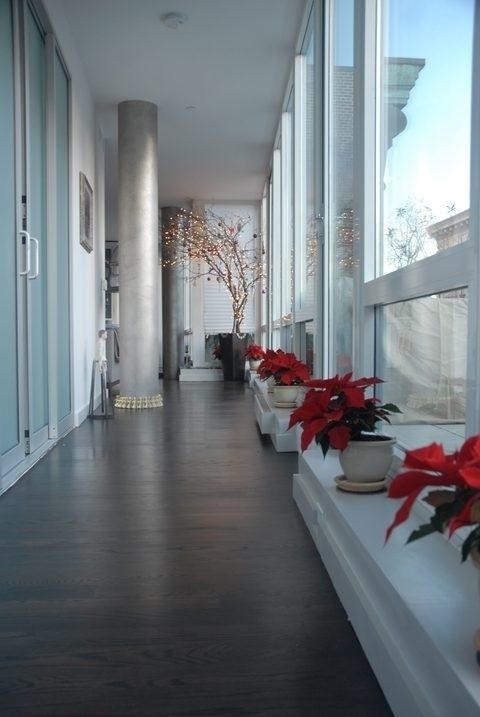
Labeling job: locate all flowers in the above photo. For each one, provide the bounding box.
[383,434,479,571]
[285,371,404,460]
[244,343,266,360]
[159,204,269,333]
[257,348,313,386]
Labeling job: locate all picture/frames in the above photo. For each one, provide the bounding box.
[79,170,94,255]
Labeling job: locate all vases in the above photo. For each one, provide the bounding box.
[219,332,255,381]
[334,434,397,493]
[250,360,261,370]
[267,385,300,408]
